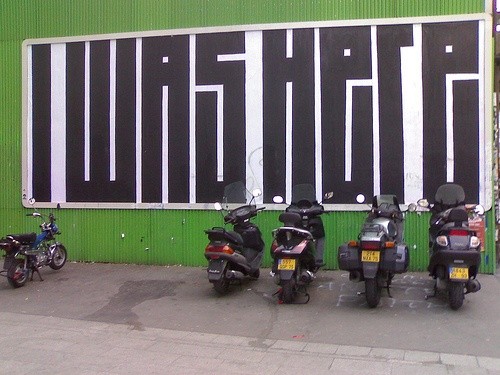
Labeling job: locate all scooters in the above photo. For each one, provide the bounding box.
[268,191,335,304]
[203,182,268,294]
[418,183,488,311]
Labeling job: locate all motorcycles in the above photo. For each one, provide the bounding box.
[0,197,68,289]
[338,194,417,308]
